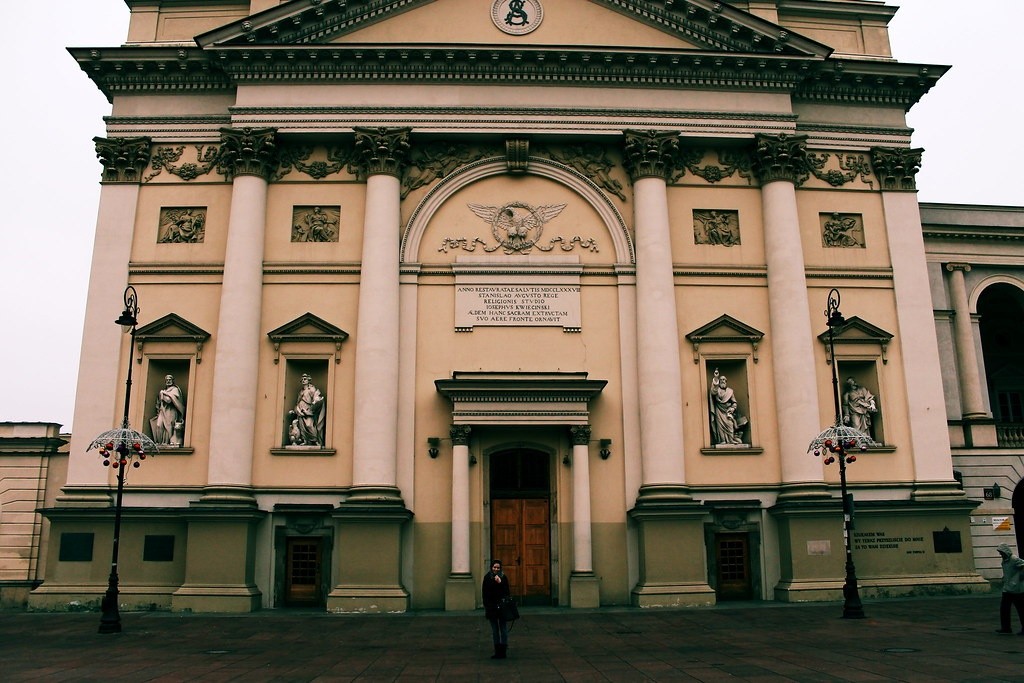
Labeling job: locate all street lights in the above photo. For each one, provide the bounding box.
[806,287,878,619]
[86,285,160,633]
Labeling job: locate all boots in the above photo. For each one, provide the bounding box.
[491,643,508,659]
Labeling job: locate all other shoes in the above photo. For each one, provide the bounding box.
[1017,630,1024,635]
[995,628,1012,634]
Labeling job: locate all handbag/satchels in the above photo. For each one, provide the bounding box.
[498,597,520,622]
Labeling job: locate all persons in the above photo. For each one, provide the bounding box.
[702,210,733,245]
[165,209,203,243]
[843,377,883,451]
[287,371,325,447]
[708,366,744,445]
[995,543,1024,636]
[303,206,338,242]
[819,212,857,248]
[148,375,184,448]
[482,560,511,658]
